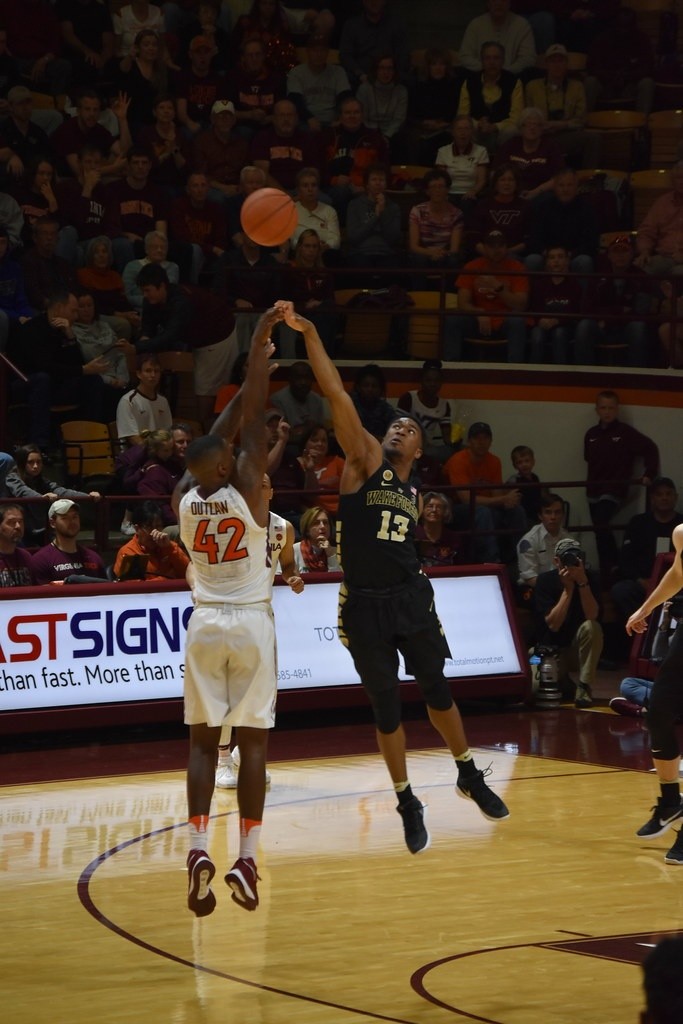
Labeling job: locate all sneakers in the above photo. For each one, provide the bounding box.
[637,794,683,839]
[574,688,592,706]
[120,520,137,535]
[186,849,217,917]
[665,824,683,864]
[455,760,510,821]
[608,696,648,718]
[397,795,428,855]
[224,857,261,910]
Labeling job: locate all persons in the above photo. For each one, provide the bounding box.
[0,0,683,718]
[276,289,511,854]
[169,305,275,918]
[625,522,683,866]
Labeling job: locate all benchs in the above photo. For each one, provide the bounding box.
[328,110,683,356]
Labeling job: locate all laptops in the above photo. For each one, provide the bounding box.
[117,554,151,581]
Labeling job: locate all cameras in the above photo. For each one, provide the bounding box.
[558,550,586,567]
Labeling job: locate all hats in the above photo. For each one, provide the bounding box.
[468,422,492,439]
[652,476,676,488]
[545,44,566,58]
[264,407,287,423]
[423,359,442,370]
[485,229,506,243]
[211,100,234,115]
[48,499,80,520]
[555,538,582,557]
[608,236,632,251]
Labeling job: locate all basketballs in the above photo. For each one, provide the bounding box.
[240,186,298,247]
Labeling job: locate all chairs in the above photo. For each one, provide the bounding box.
[129,350,197,375]
[60,421,117,478]
